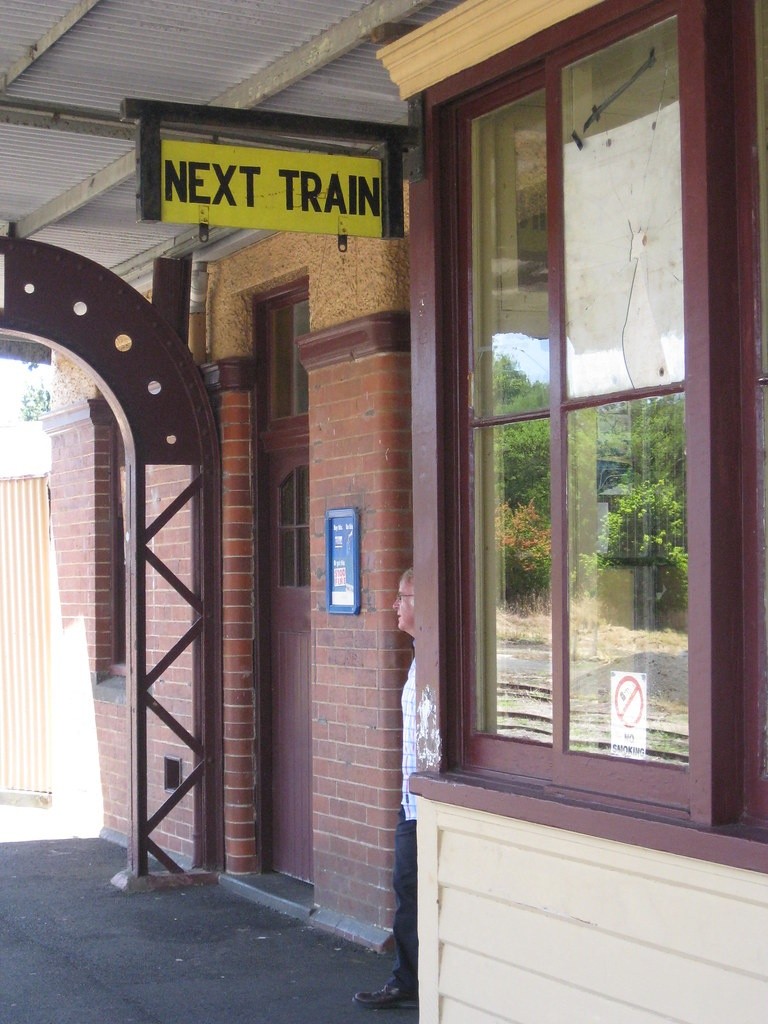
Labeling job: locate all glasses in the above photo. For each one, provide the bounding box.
[395,592,413,600]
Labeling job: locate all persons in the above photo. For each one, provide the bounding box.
[353,563,417,1008]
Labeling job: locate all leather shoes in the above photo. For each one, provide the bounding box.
[353,983,419,1009]
